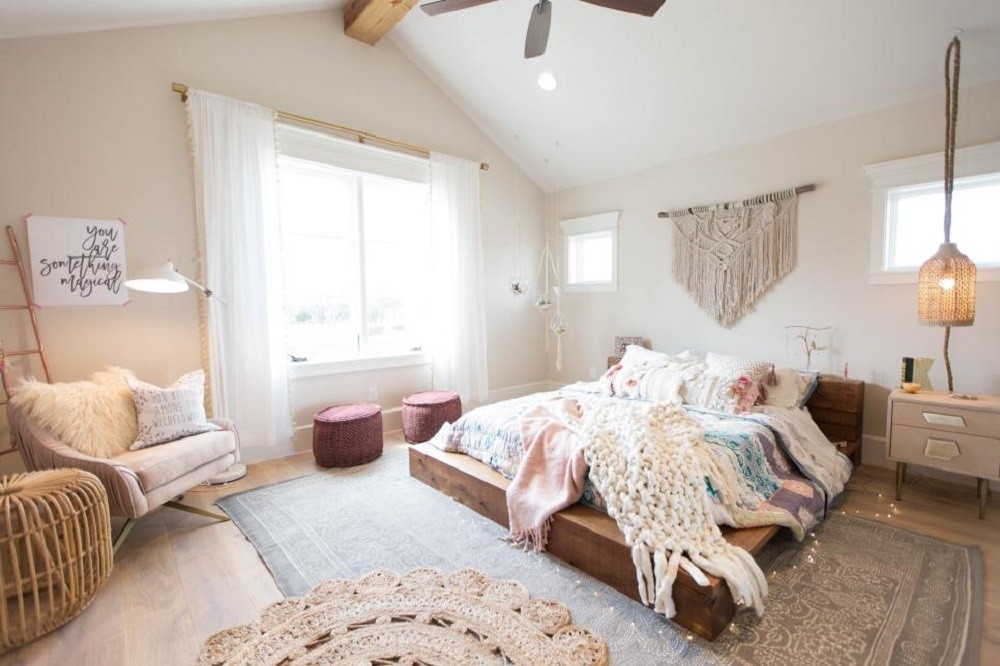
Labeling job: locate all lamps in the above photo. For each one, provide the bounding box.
[916,38,975,393]
[509,240,569,369]
[124,260,246,484]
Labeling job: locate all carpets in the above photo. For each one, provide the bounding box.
[215,454,981,666]
[195,568,606,666]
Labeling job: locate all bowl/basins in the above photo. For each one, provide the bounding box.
[901,382,923,392]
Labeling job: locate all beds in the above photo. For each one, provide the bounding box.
[409,357,864,642]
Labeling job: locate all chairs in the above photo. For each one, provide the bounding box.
[7,363,242,559]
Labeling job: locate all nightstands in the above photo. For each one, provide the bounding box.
[886,388,1000,521]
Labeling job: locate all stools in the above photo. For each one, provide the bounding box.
[311,403,383,466]
[401,392,462,441]
[0,465,117,654]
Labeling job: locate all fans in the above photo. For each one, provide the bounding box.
[420,0,665,58]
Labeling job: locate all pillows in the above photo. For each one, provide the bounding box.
[123,369,223,451]
[613,344,774,412]
